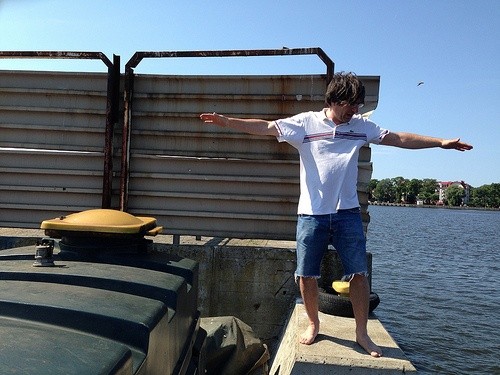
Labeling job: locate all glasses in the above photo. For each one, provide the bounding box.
[346,102,365,109]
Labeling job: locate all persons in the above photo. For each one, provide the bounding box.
[200,70,474,357]
